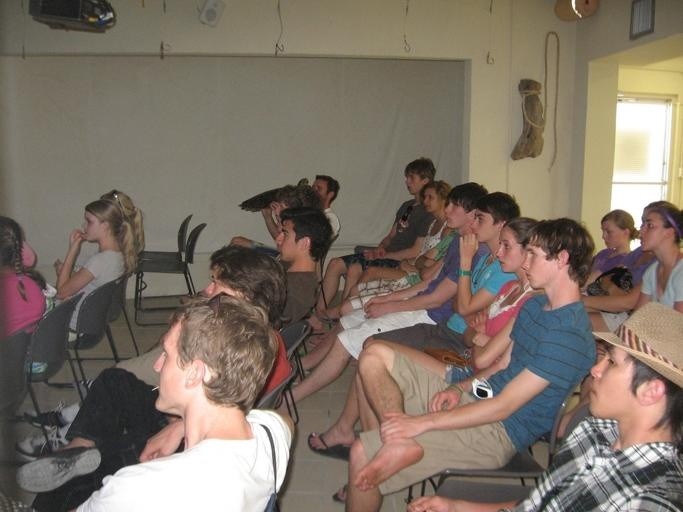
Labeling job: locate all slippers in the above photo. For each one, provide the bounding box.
[333,483,350,501]
[308,432,349,460]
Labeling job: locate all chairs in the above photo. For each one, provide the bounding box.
[28,291,84,458]
[318,232,337,333]
[255,360,297,410]
[134,213,207,327]
[106,269,139,369]
[407,379,582,502]
[435,404,591,503]
[1,329,52,457]
[68,278,122,394]
[278,319,312,425]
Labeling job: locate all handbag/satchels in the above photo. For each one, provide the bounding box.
[425,348,470,365]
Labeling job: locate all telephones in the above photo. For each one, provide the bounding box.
[1,52,472,267]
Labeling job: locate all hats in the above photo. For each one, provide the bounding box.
[591,301,683,388]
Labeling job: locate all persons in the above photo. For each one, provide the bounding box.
[632,203,683,312]
[228,175,342,336]
[580,208,640,294]
[13,242,286,512]
[289,158,537,460]
[13,207,332,460]
[405,301,683,512]
[581,251,673,355]
[333,216,595,512]
[0,189,146,375]
[74,294,294,512]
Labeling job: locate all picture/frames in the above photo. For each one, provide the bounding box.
[629,1,655,39]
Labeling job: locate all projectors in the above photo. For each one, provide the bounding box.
[29,0,116,33]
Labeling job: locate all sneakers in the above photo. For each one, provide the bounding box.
[25,401,68,430]
[16,448,102,492]
[15,428,67,460]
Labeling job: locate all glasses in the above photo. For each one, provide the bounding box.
[402,206,413,221]
[472,378,493,399]
